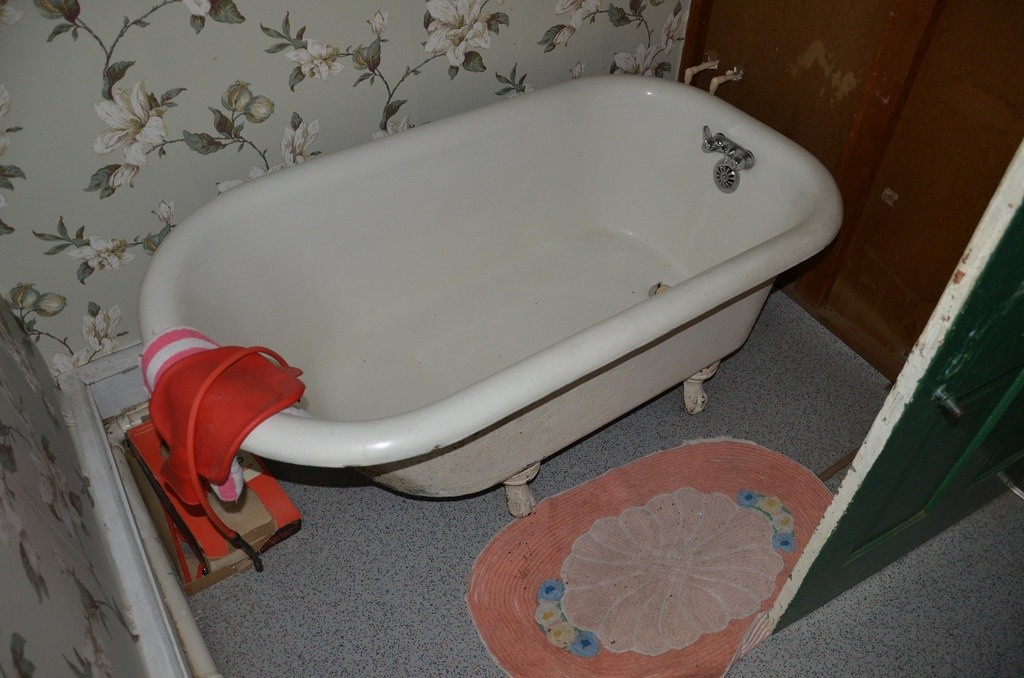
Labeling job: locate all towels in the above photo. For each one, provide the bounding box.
[138,325,315,503]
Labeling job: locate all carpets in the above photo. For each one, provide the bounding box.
[462,436,835,678]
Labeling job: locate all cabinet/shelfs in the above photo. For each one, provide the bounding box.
[675,0,1022,392]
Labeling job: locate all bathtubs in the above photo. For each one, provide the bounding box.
[140,75,844,516]
